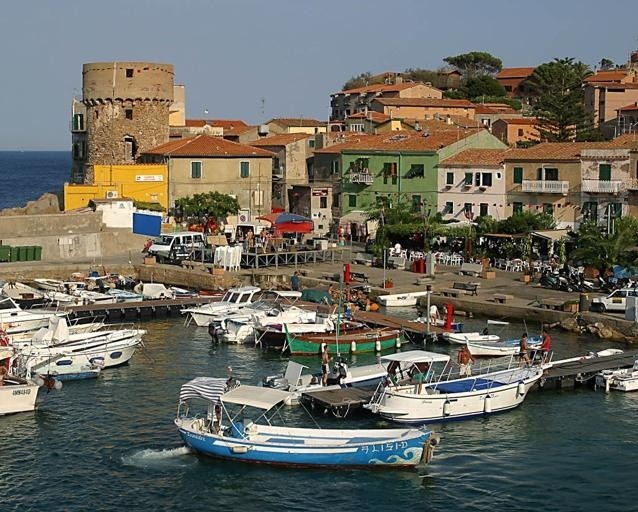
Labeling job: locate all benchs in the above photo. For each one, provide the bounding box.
[439,282,478,298]
[493,293,514,305]
[540,299,565,310]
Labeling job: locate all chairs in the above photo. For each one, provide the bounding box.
[493,245,553,273]
[387,246,464,267]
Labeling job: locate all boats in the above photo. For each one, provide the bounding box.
[133,280,172,300]
[284,328,410,356]
[594,361,638,394]
[362,349,545,425]
[3,272,147,416]
[180,285,261,327]
[462,335,544,355]
[169,376,439,479]
[378,290,433,308]
[415,311,464,333]
[255,312,367,350]
[211,289,315,344]
[442,330,501,347]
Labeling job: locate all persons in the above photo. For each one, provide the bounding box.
[394,240,403,257]
[234,226,275,255]
[458,345,476,379]
[321,345,334,385]
[337,235,346,246]
[409,362,428,394]
[289,271,300,300]
[326,283,380,336]
[428,304,440,328]
[141,238,152,253]
[331,221,338,239]
[530,329,551,361]
[144,239,149,247]
[338,225,345,236]
[518,332,531,367]
[387,360,404,387]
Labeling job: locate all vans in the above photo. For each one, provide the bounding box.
[149,231,207,258]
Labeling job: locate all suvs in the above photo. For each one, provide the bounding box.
[589,289,637,313]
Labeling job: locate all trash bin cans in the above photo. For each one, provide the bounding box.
[0,245,42,262]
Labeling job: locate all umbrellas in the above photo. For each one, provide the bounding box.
[254,212,315,225]
[274,221,312,241]
